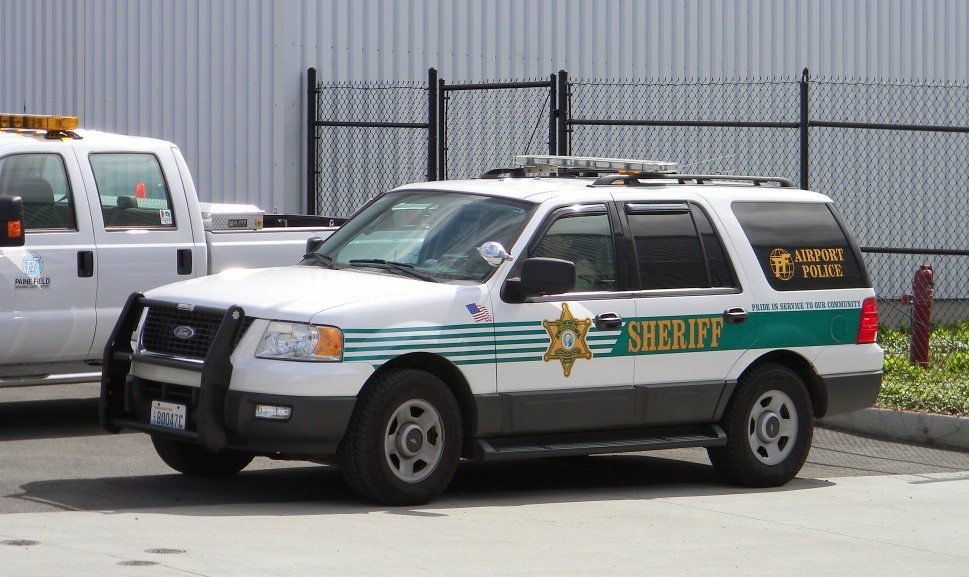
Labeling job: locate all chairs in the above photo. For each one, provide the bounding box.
[17,176,64,230]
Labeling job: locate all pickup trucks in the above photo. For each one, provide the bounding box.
[0,111,430,389]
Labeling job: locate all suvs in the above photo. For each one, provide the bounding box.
[96,153,885,510]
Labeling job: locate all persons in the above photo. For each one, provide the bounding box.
[533,222,602,291]
